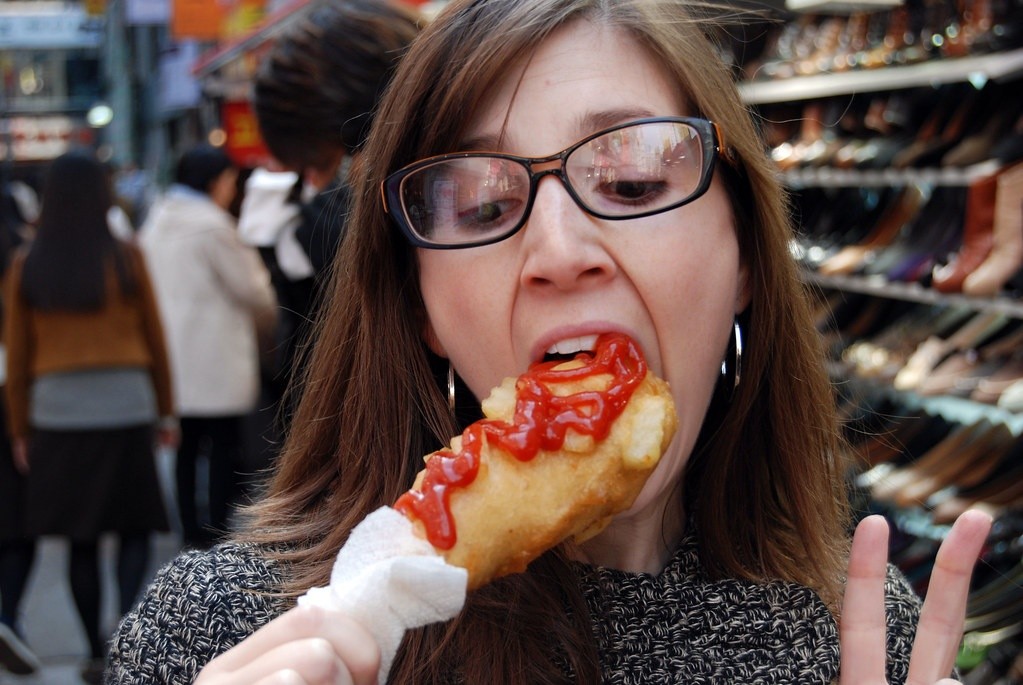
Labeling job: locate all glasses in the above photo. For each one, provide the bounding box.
[378,114,738,250]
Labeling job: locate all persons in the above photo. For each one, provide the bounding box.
[105,0,995,685]
[0,1,430,685]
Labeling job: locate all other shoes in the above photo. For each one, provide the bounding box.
[80,655,107,685]
[0,622,41,676]
[727,0,1023,685]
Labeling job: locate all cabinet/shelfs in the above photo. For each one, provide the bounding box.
[732,49,1023,559]
